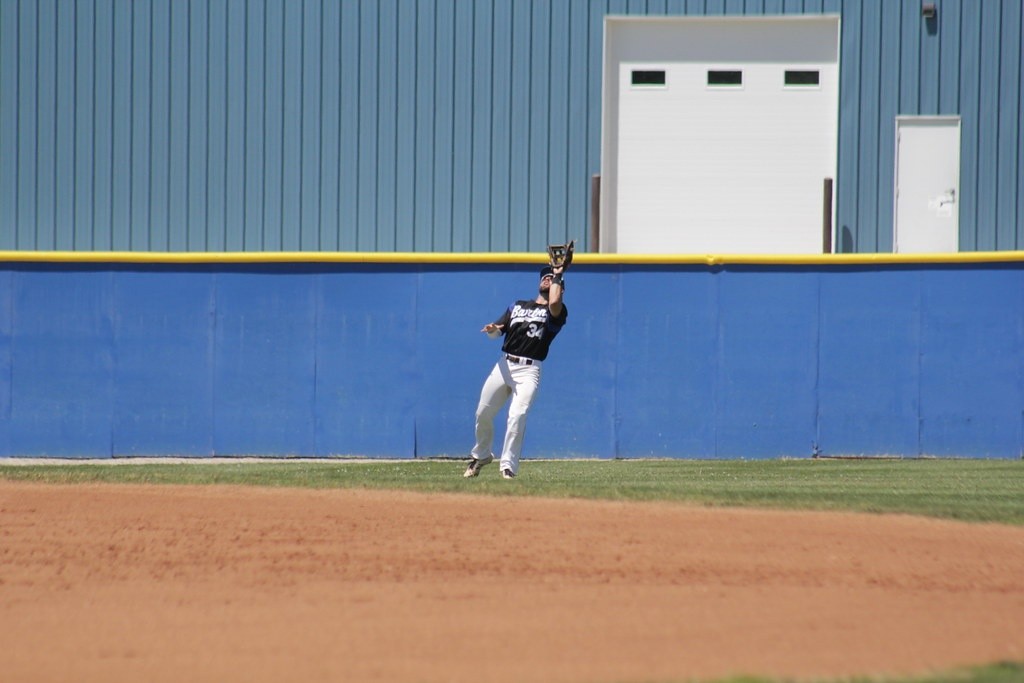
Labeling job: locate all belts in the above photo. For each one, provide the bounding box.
[506,355,532,365]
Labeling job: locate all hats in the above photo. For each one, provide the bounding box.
[540,267,564,288]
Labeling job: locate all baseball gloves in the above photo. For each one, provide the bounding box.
[548,240,575,275]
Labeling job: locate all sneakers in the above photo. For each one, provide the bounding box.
[463,453,494,478]
[503,469,514,479]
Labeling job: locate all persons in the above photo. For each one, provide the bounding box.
[463,240,574,479]
